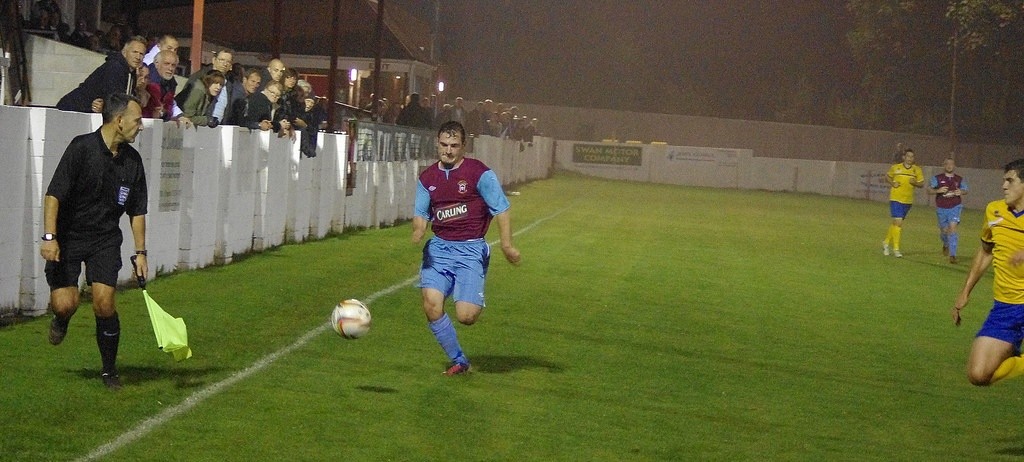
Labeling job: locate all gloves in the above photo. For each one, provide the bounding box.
[207,116,219,129]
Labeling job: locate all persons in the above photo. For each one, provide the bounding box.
[953,160,1024,386]
[411,121,521,376]
[0,0,543,144]
[40,90,148,387]
[883,147,926,258]
[925,158,969,264]
[892,143,905,163]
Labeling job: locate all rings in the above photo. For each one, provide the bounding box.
[94,107,96,109]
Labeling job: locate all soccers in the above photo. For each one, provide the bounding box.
[331,299,371,340]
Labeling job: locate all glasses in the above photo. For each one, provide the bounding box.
[216,57,233,65]
[267,89,280,99]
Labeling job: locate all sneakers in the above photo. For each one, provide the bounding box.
[48,315,68,344]
[101,370,122,389]
[442,363,468,375]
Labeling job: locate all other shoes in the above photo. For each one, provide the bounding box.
[883,241,889,255]
[893,251,902,258]
[942,247,949,256]
[949,256,958,264]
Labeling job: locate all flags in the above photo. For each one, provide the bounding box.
[142,290,193,362]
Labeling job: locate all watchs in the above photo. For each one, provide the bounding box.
[136,250,148,256]
[41,233,57,241]
